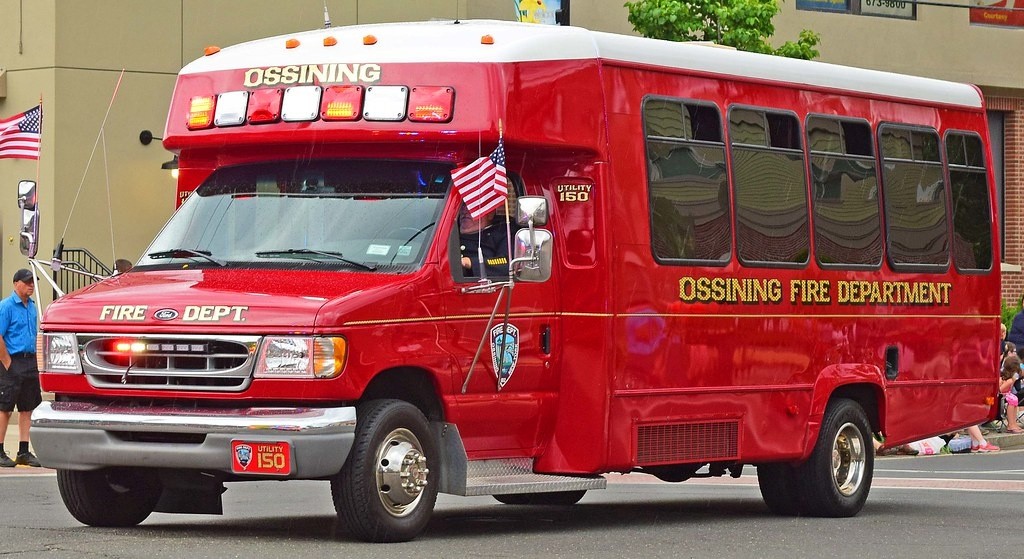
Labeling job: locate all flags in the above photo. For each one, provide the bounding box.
[0,105,42,160]
[451,138,508,221]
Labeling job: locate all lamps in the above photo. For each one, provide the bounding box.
[140,131,179,181]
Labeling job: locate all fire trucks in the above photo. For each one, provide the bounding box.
[7,20,1002,545]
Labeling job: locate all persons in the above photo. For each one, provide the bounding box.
[0,269,42,467]
[459,198,523,277]
[872,296,1024,456]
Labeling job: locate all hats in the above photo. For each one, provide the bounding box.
[13,268,40,282]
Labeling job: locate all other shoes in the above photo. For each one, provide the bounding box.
[979,427,990,435]
[1006,427,1024,433]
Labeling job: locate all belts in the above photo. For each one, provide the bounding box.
[12,353,36,358]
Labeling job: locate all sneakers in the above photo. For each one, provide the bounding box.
[971,444,979,453]
[978,442,1000,453]
[897,445,919,455]
[876,445,896,456]
[0,451,16,467]
[15,452,40,466]
